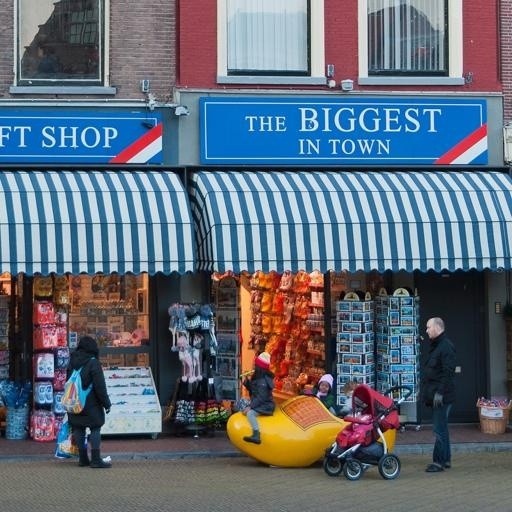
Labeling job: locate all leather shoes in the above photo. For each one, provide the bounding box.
[426,464,444,472]
[442,461,451,468]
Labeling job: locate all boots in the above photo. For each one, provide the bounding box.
[244,431,261,445]
[77,446,90,467]
[90,449,112,468]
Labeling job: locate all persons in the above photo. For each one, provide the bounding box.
[299,374,336,417]
[61,337,112,468]
[242,352,274,445]
[421,316,457,472]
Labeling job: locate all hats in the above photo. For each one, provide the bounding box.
[318,373,334,389]
[256,352,271,368]
[168,301,218,384]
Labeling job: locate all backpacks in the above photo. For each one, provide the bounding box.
[62,357,93,415]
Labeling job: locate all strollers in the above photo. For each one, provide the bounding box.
[322,386,413,478]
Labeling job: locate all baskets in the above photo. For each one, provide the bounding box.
[477,397,511,434]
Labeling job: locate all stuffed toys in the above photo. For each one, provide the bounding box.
[168,303,218,384]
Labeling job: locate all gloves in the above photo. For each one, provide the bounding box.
[433,392,443,410]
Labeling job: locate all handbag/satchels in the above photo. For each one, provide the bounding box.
[163,377,232,427]
